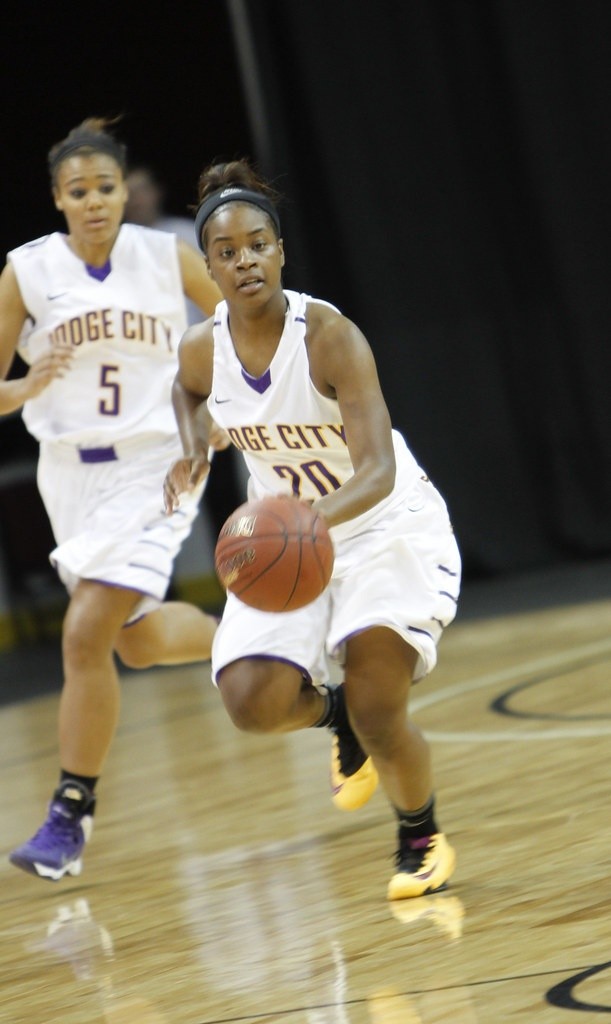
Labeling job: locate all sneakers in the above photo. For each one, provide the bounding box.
[8,780,97,882]
[327,682,380,811]
[385,832,456,900]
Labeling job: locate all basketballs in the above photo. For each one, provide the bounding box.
[214,497,335,614]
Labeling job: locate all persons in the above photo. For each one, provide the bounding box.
[0,119,225,882]
[163,160,462,900]
